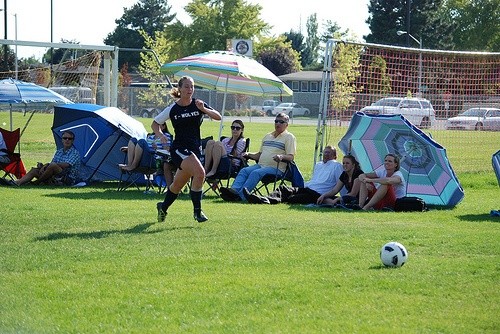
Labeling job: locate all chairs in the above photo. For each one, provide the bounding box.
[198,137,251,198]
[158,135,214,195]
[243,153,296,198]
[0,126,22,185]
[117,134,174,192]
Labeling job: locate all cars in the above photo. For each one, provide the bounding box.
[274,103,310,118]
[447,108,500,131]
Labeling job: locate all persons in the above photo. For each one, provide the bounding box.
[4,131,81,188]
[346,153,407,211]
[243,145,344,204]
[220,112,297,201]
[204,120,246,177]
[0,132,8,164]
[151,76,222,223]
[119,120,172,170]
[316,155,364,208]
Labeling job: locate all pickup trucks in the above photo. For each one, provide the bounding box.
[250,99,280,116]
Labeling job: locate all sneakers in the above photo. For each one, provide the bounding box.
[194,209,209,222]
[156,202,166,222]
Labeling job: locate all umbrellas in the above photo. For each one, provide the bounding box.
[0,77,75,132]
[51,103,148,185]
[160,51,293,141]
[338,112,465,210]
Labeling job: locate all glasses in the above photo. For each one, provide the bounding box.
[275,119,287,124]
[231,126,242,131]
[62,137,74,140]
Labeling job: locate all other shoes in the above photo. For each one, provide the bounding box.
[218,186,239,202]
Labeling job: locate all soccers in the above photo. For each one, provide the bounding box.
[379,241,409,269]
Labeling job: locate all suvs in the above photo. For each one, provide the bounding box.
[359,97,436,129]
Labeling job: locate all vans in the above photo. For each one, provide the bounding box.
[48,86,92,103]
